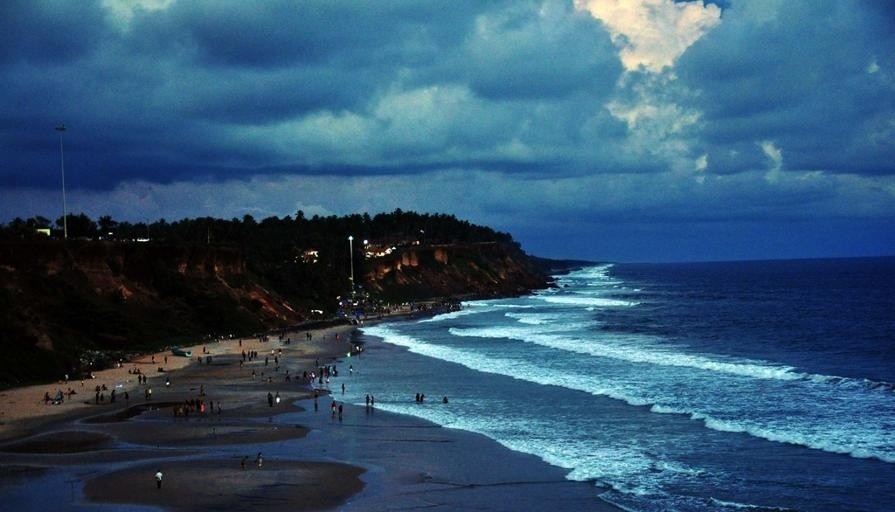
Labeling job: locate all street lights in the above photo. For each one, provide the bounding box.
[347,236,356,300]
[53,125,70,236]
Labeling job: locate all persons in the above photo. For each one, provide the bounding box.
[42,303,448,489]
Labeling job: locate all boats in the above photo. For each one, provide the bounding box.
[172,348,192,356]
